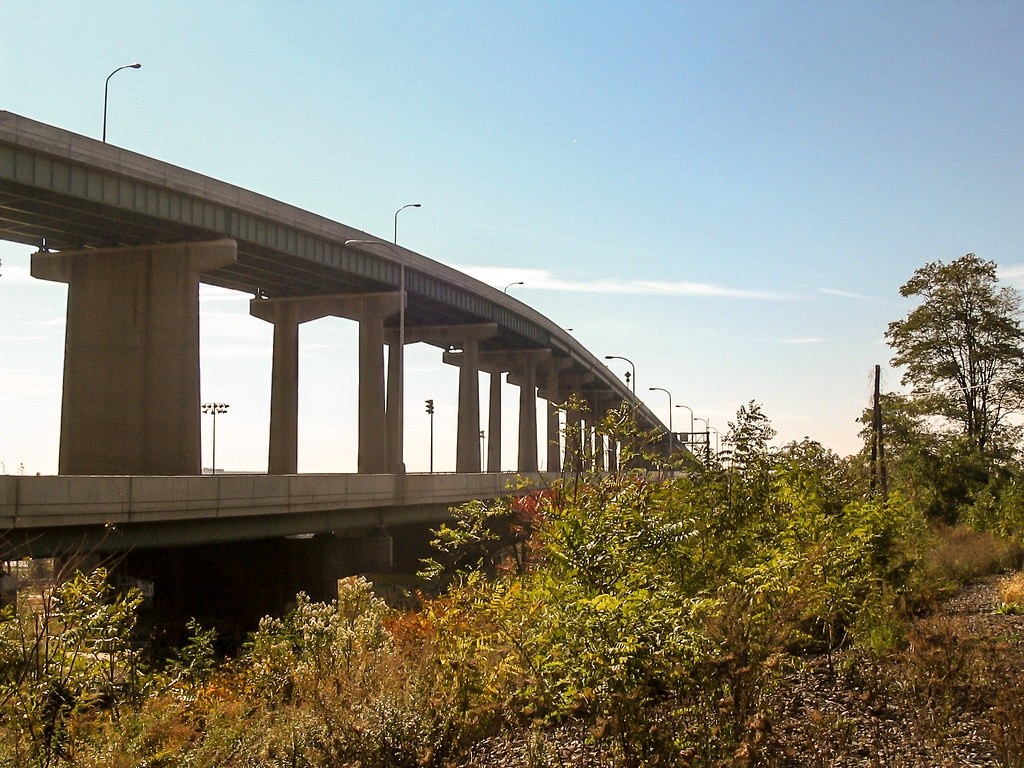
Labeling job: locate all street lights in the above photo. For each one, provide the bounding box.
[101,63,142,144]
[676,405,694,456]
[706,426,719,461]
[395,204,421,244]
[342,238,405,477]
[694,417,708,441]
[203,401,230,475]
[425,399,434,474]
[712,431,723,471]
[648,387,672,467]
[480,430,486,473]
[604,355,637,470]
